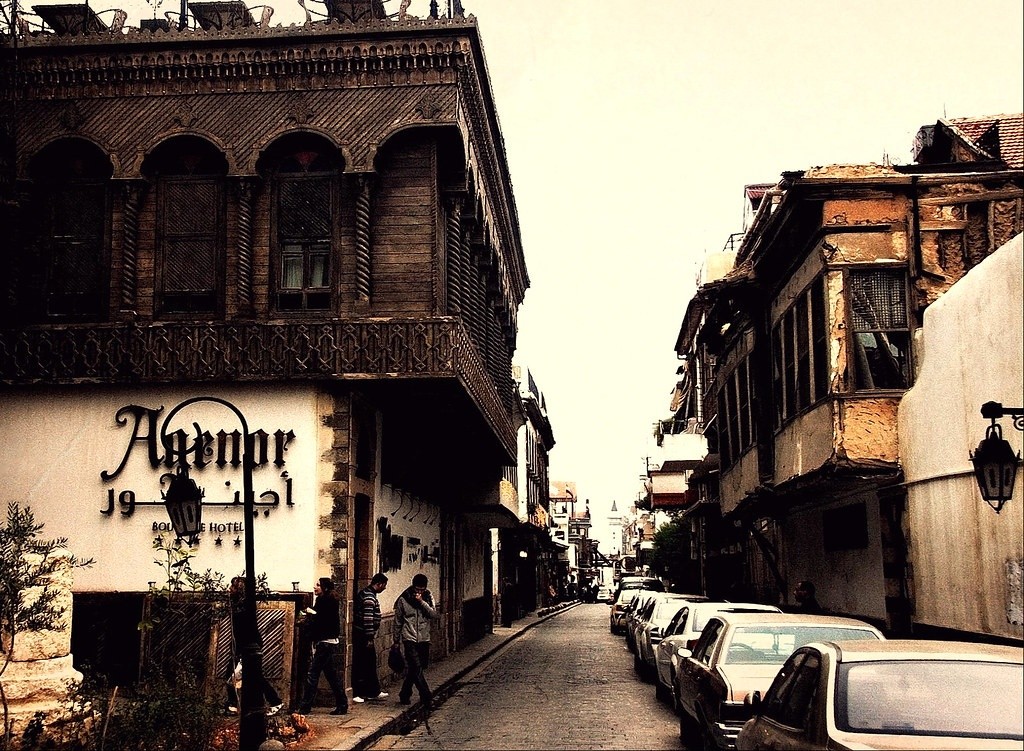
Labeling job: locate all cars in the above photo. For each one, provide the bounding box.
[653,602,785,717]
[595,575,711,683]
[733,637,1024,751]
[676,611,887,751]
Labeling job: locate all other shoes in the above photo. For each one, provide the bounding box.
[219,706,243,715]
[425,698,435,711]
[367,692,390,700]
[330,708,350,716]
[399,695,412,706]
[266,702,286,716]
[289,707,313,715]
[352,696,366,703]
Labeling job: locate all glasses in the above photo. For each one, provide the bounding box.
[414,586,427,592]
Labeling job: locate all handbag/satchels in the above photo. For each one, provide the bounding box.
[387,642,405,675]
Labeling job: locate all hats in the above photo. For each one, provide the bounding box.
[320,576,336,596]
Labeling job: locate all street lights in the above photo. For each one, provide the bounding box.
[156,393,268,750]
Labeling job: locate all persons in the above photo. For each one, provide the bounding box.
[392,574,437,706]
[353,573,389,703]
[794,581,822,615]
[289,577,349,715]
[548,582,599,606]
[500,576,513,628]
[224,577,284,716]
